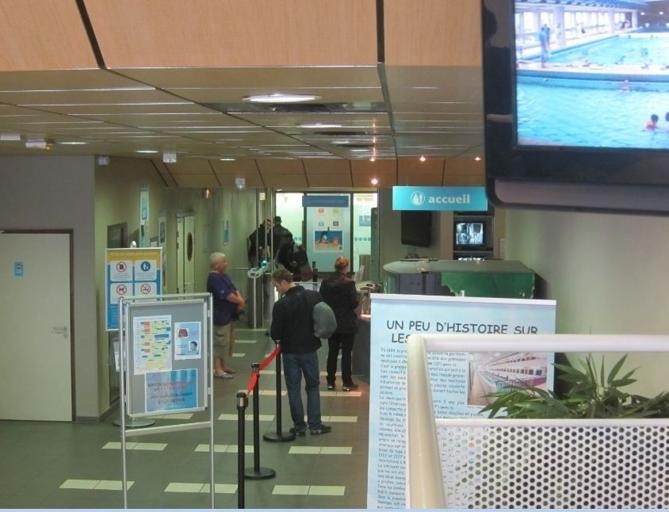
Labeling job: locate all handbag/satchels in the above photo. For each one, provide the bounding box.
[311,300,339,341]
[300,264,312,282]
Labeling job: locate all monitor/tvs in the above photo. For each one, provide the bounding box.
[454,215,494,261]
[481,0,669,187]
[400,211,432,247]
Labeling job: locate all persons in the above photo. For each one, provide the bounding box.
[316,232,329,251]
[267,214,296,270]
[269,267,334,436]
[189,339,197,351]
[318,254,361,391]
[205,250,246,380]
[330,236,340,251]
[514,0,669,134]
[248,218,273,300]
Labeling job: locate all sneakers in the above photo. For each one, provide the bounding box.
[327,383,359,390]
[310,424,332,434]
[215,368,236,379]
[289,426,306,437]
